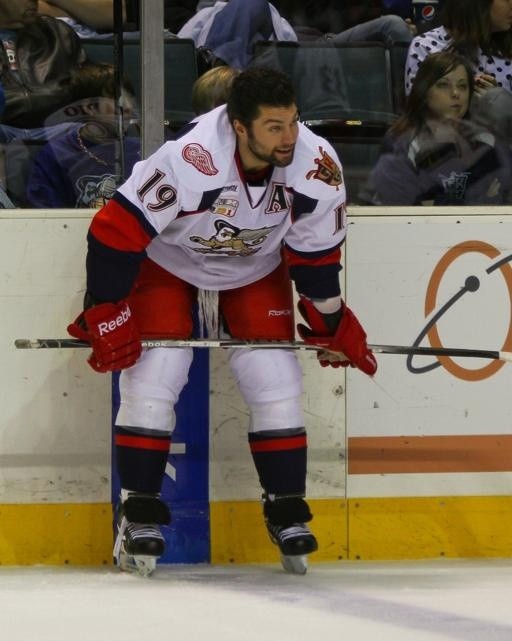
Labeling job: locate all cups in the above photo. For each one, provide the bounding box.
[412,1,440,34]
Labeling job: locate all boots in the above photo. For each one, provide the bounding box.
[115,486,171,559]
[261,492,317,558]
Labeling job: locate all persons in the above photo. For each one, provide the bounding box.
[67,66,378,556]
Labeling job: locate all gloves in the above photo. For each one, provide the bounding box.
[67,299,142,373]
[297,298,377,376]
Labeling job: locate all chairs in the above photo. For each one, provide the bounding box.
[81,36,404,134]
